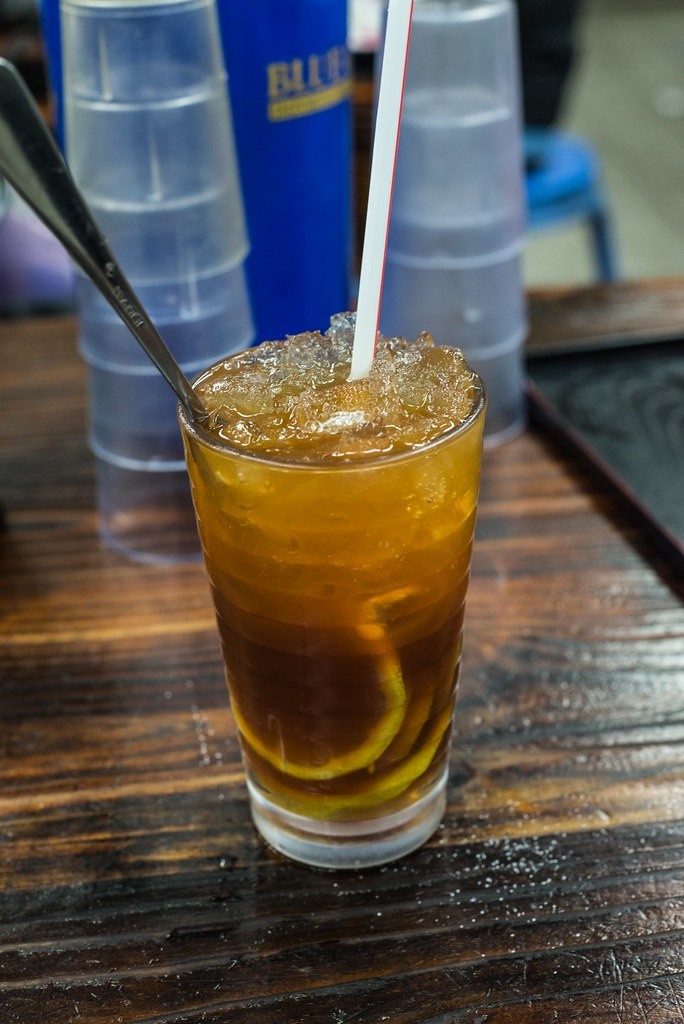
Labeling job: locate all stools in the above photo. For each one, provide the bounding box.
[522,124,618,290]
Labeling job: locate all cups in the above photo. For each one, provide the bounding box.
[40,0,530,565]
[178,334,483,870]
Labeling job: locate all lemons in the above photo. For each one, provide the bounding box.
[224,586,457,815]
